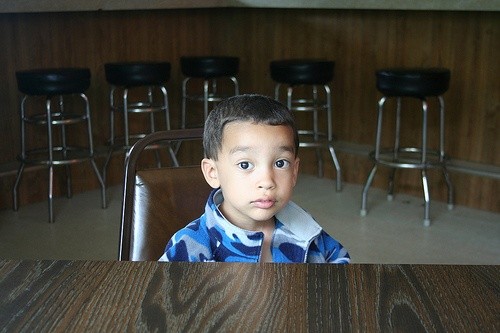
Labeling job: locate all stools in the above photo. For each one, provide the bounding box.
[269,58,343,193]
[360,67,455,227]
[13,68,108,222]
[171,55,240,167]
[102,59,181,182]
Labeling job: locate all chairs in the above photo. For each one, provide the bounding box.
[117,129,214,261]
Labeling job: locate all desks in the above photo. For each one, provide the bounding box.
[0,258,500,333]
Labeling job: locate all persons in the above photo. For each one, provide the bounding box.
[158,95,350,263]
